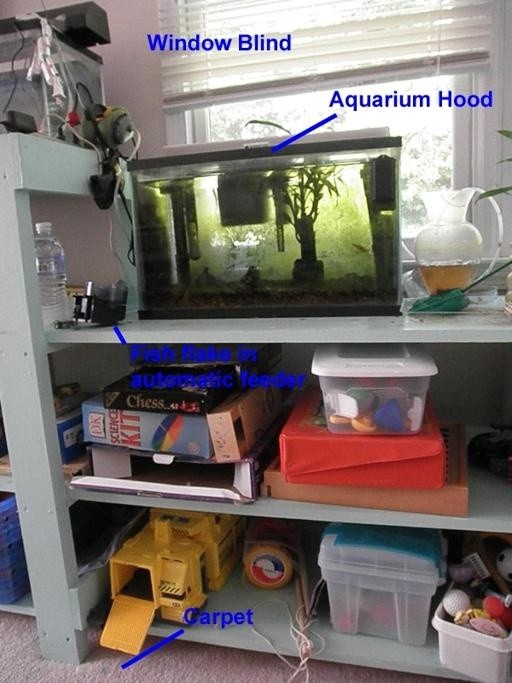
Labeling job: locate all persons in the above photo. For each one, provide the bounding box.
[99,504,243,656]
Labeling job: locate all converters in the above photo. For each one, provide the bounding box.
[4,110,38,133]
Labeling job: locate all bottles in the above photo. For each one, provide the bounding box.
[31,220,72,326]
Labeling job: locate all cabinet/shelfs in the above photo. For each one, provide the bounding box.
[47,301,512,682]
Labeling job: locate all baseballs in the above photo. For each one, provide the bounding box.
[442,589,470,617]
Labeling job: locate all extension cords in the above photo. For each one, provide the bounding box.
[30,130,81,148]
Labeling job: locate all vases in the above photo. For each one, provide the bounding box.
[414,189,483,296]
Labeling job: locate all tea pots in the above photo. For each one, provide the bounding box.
[416,186,504,299]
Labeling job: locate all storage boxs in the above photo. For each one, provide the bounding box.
[316,523,448,647]
[312,342,439,435]
[432,582,512,683]
[55,343,292,507]
[0,12,105,150]
[278,382,447,491]
